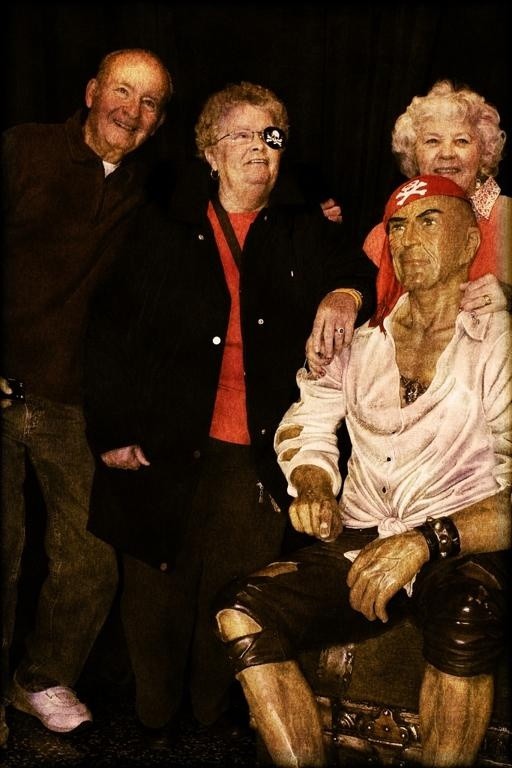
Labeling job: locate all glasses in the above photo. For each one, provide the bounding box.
[210,125,288,150]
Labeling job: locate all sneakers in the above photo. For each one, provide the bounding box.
[2,674,94,735]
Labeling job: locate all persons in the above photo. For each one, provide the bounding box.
[210,175,512,768]
[0,49,343,748]
[305,79,512,380]
[80,82,375,749]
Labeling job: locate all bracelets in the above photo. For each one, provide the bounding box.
[332,287,364,311]
[414,516,461,561]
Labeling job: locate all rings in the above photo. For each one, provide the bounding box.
[334,328,344,334]
[483,295,491,305]
